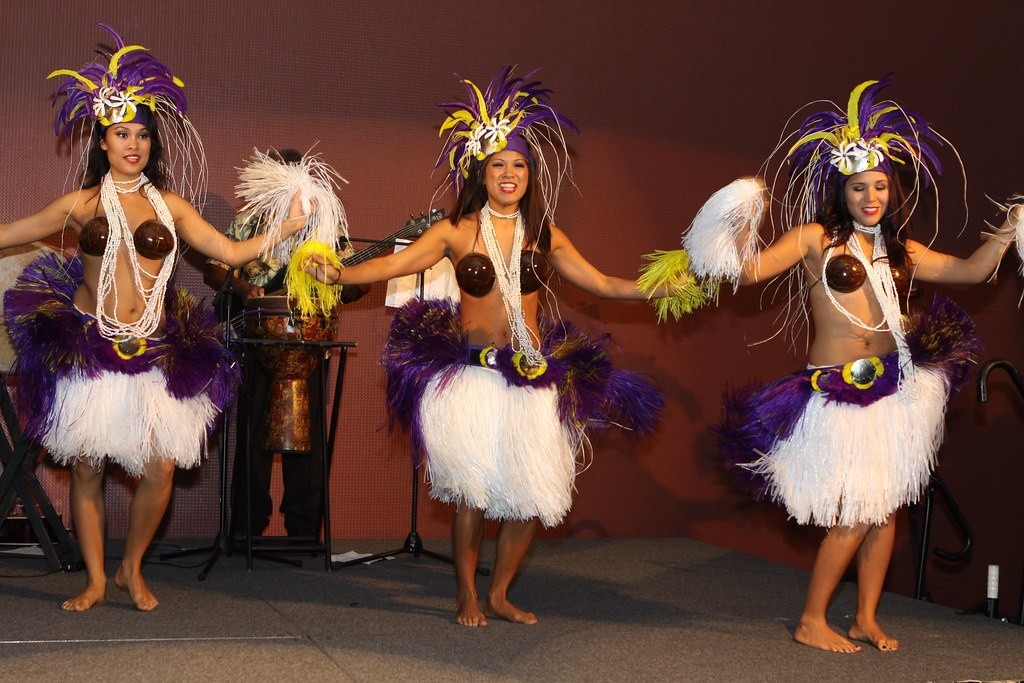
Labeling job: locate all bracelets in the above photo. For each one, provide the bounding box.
[328,266,341,286]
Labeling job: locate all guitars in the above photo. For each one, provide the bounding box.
[208,207,447,367]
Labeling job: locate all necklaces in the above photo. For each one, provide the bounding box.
[821,220,917,399]
[96,167,177,342]
[480,200,542,366]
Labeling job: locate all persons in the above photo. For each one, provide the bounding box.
[204,148,372,554]
[726,130,1023,652]
[0,93,317,612]
[297,125,717,629]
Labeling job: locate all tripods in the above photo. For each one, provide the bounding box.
[160,221,304,581]
[327,235,492,579]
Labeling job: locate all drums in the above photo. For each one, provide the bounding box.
[245,306,339,452]
